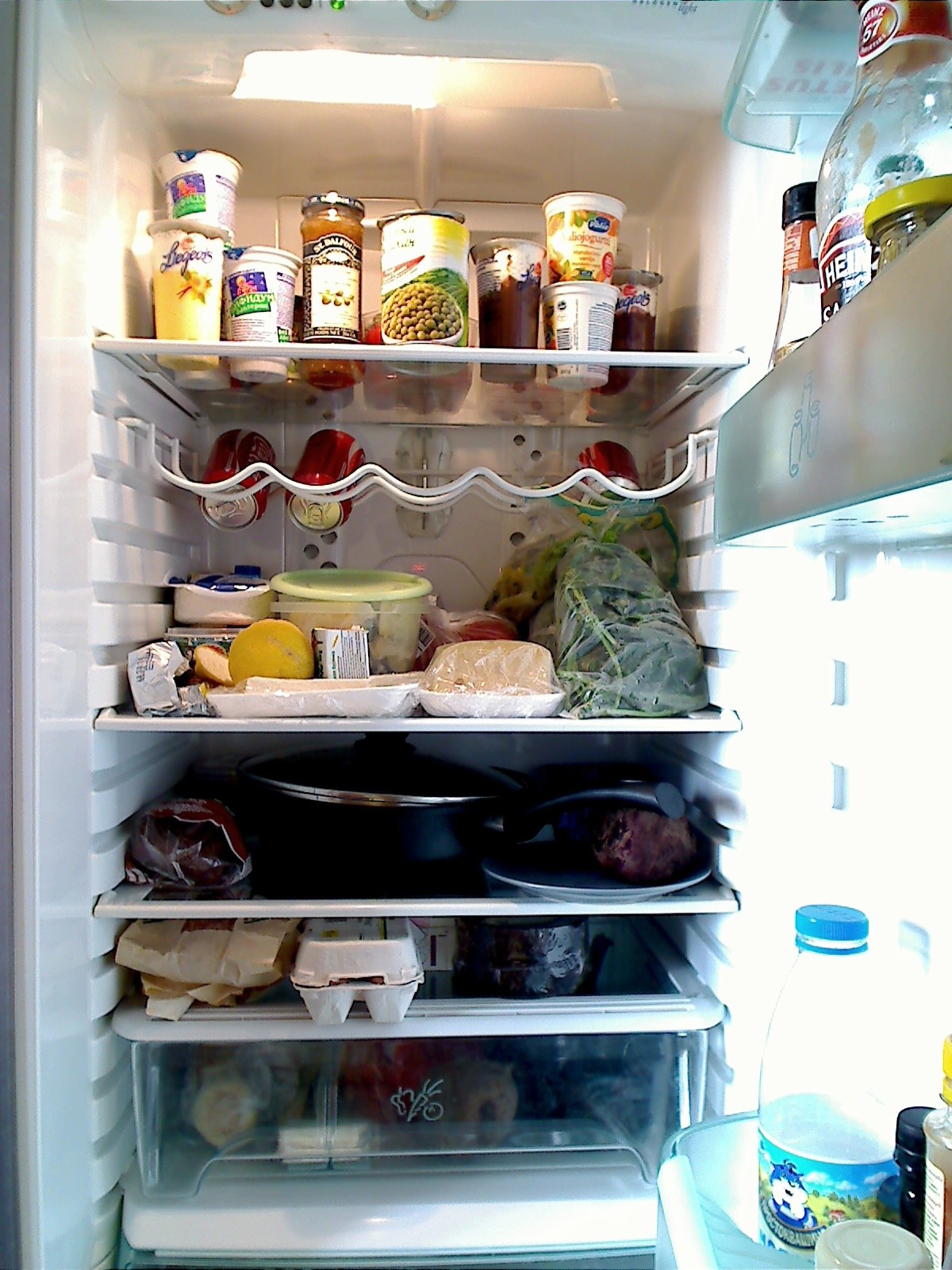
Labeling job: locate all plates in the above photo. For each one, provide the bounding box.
[204,683,419,719]
[417,688,566,717]
[476,855,714,901]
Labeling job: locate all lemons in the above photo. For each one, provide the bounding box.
[222,613,315,692]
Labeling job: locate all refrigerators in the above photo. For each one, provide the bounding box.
[14,0,952,1270]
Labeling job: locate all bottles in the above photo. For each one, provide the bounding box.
[768,180,828,367]
[815,0,952,325]
[756,893,951,1269]
